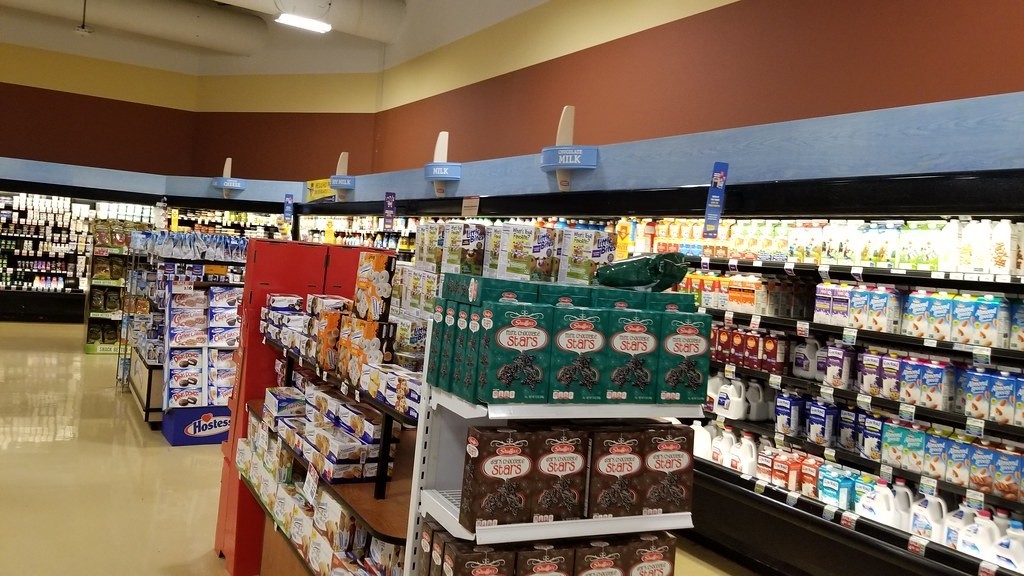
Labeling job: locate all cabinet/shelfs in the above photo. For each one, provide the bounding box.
[0,192,130,355]
[216,239,695,576]
[298,211,1024,574]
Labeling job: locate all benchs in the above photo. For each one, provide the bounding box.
[118,233,246,432]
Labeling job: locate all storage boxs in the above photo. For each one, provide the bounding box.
[138,227,1024,576]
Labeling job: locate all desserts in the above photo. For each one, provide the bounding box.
[225,295,242,346]
[179,358,198,406]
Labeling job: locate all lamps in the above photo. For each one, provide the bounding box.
[272,0,332,34]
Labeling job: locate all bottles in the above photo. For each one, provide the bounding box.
[680,333,835,477]
[861,477,1024,574]
[33,275,63,290]
